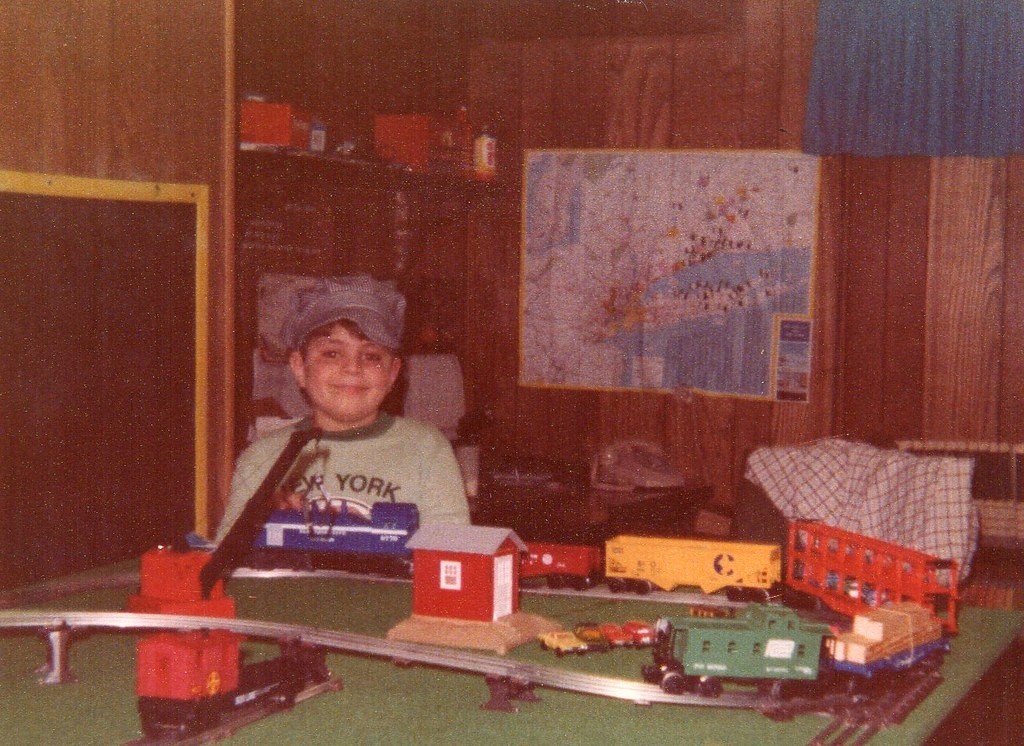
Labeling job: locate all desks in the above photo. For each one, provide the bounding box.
[469,468,721,534]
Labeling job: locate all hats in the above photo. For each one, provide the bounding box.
[281,275,408,350]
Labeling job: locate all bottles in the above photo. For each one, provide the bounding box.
[451,101,473,178]
[473,122,498,184]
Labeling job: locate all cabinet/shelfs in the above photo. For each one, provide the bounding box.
[239,147,505,434]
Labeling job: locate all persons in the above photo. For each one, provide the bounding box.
[220,275,472,541]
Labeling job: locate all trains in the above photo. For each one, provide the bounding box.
[252,502,947,701]
